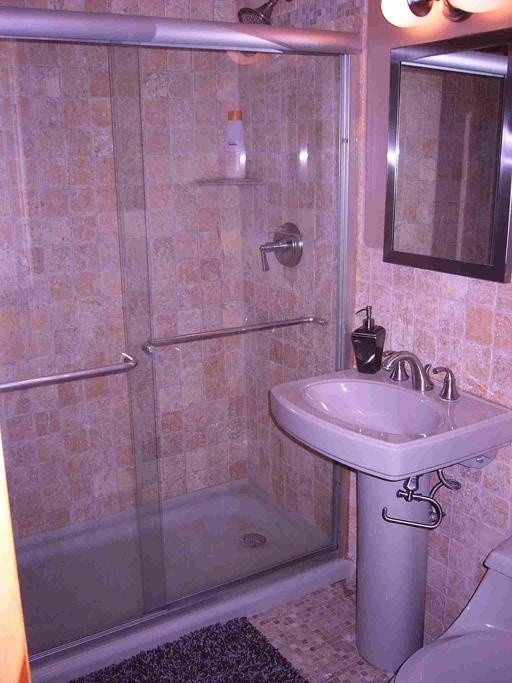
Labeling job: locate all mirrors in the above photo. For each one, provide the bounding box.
[382,27,512,283]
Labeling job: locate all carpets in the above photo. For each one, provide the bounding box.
[69,616,309,683]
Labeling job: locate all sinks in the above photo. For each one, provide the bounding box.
[269,369,512,481]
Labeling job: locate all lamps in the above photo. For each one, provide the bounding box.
[381,0,498,27]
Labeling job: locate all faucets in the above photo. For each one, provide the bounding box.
[383,351,433,392]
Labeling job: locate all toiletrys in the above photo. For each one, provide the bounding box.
[351,306,386,374]
[223,110,247,179]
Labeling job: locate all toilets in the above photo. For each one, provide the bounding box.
[387,536,512,683]
